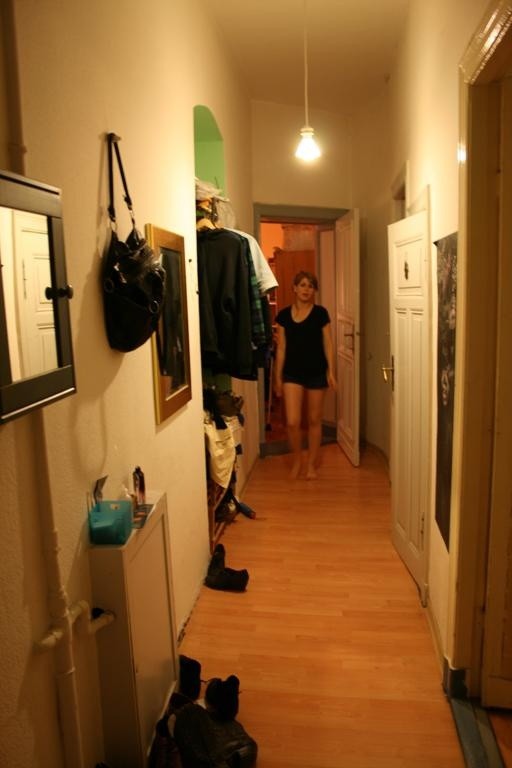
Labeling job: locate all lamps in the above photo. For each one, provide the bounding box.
[288,1,332,175]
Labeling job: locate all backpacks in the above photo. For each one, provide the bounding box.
[147,703,256,768]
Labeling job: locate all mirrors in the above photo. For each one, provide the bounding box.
[147,224,192,426]
[1,171,76,424]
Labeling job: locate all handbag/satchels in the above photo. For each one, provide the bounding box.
[97,132,166,352]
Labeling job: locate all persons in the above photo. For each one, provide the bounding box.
[274,271,337,480]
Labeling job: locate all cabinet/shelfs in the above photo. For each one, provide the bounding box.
[272,250,316,328]
[93,488,195,767]
